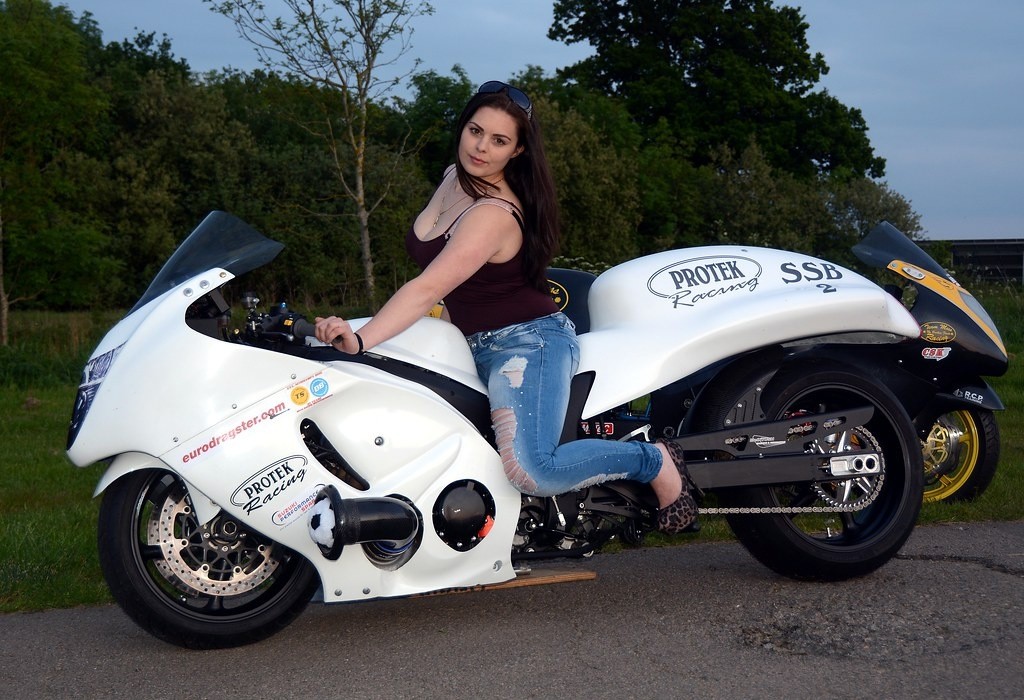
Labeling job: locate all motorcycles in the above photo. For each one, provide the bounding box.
[65,208,931,651]
[843,219,1011,509]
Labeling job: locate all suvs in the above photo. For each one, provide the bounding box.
[960,262,1017,292]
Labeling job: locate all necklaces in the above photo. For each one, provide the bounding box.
[433,175,505,228]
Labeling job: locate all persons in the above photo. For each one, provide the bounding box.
[316,80,708,536]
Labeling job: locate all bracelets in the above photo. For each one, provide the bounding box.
[354,332,363,356]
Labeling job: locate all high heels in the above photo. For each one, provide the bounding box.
[654,438,704,534]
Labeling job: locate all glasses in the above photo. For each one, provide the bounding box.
[478,80,533,122]
